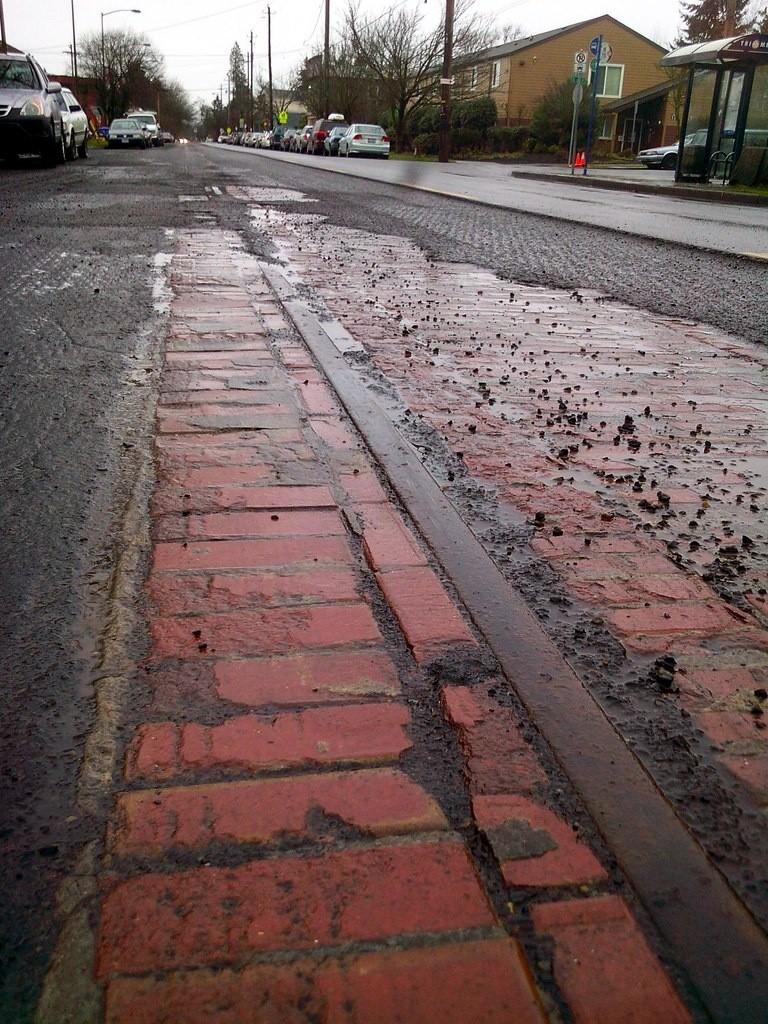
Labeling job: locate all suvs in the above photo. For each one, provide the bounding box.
[306,119,349,156]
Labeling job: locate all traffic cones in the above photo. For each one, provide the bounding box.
[581,152,586,166]
[574,153,582,166]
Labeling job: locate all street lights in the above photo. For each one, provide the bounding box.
[119,43,151,78]
[101,9,141,126]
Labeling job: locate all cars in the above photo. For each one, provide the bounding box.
[218,136,227,143]
[279,125,313,153]
[108,119,152,149]
[0,53,65,166]
[57,88,90,160]
[162,133,174,143]
[338,124,390,159]
[126,114,158,144]
[636,134,695,170]
[226,132,284,151]
[322,127,349,156]
